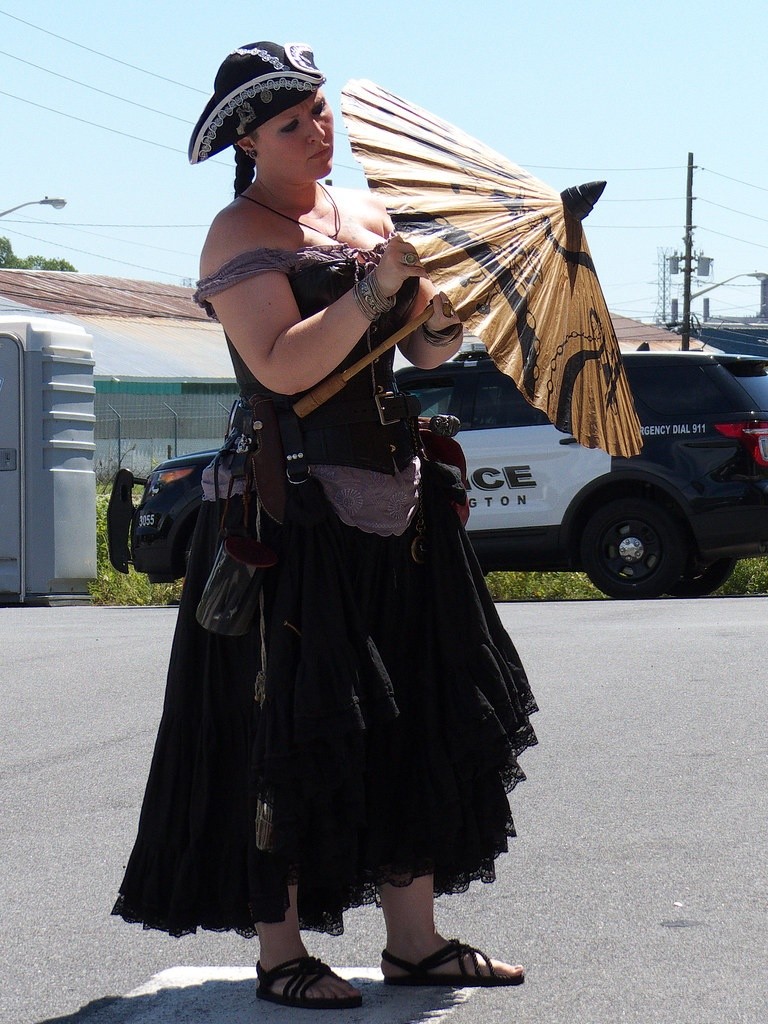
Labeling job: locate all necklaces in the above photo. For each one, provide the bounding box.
[239,179,342,239]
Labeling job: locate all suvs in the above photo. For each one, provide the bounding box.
[106,342,766,600]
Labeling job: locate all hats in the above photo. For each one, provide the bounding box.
[187,40,328,166]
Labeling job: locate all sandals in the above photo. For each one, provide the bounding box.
[381,939,525,988]
[255,956,363,1009]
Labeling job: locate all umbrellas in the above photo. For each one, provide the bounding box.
[292,78,643,458]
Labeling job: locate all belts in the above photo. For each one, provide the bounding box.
[232,390,422,436]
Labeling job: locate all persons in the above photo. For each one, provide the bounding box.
[187,41,524,1009]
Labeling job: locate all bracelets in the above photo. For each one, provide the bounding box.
[421,304,463,347]
[354,269,396,321]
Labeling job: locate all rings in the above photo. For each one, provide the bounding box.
[404,253,418,267]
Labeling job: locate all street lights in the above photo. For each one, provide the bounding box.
[689,271,768,304]
[0,195,67,219]
